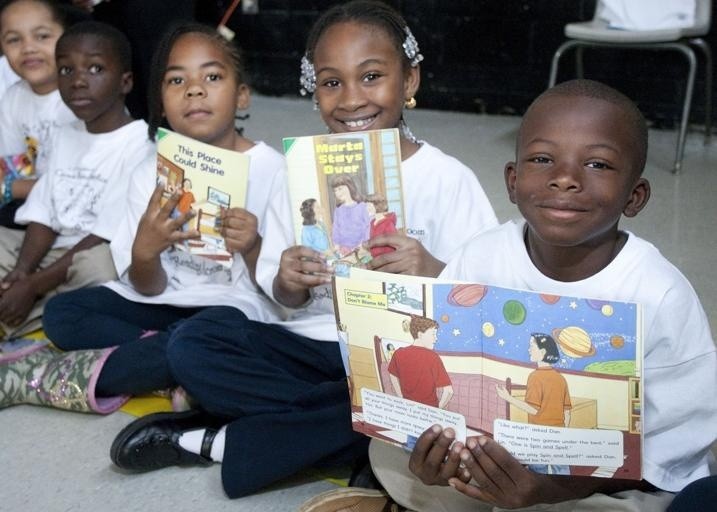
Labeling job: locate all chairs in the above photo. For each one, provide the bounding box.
[546,0,717,173]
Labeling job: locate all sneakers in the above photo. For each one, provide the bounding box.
[298,487,415,512]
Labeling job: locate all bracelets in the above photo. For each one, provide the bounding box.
[4,169,14,206]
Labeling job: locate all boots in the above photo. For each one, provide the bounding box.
[0,342,131,415]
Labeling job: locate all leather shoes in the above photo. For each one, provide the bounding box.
[109,409,230,470]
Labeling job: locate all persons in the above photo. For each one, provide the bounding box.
[110,1,499,501]
[0,22,160,341]
[0,20,288,414]
[298,79,715,512]
[1,0,141,228]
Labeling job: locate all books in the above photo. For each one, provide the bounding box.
[276,127,409,274]
[157,129,254,274]
[330,268,648,491]
